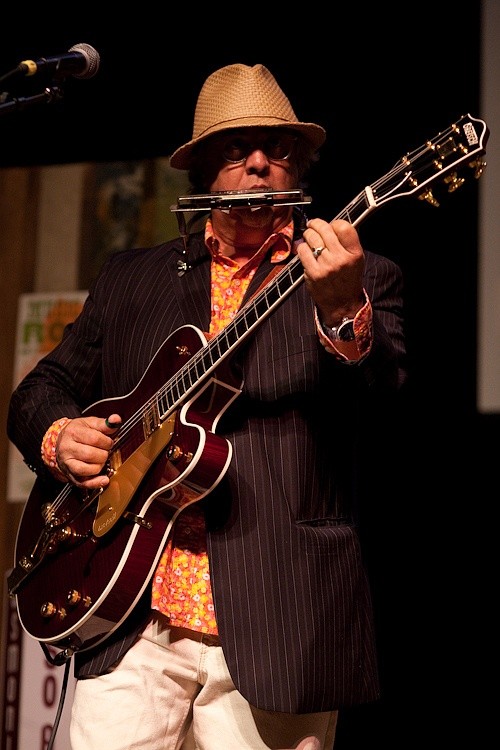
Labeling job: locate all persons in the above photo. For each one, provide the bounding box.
[4,63,410,750]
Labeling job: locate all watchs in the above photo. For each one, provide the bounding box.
[321,317,369,342]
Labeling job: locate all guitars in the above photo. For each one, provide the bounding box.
[4,116,491,652]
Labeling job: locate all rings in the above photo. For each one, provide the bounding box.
[312,248,326,259]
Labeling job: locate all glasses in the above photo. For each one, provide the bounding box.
[219,133,294,161]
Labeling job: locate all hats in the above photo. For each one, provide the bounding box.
[168,64,327,171]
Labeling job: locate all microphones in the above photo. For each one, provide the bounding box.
[21,43,100,79]
